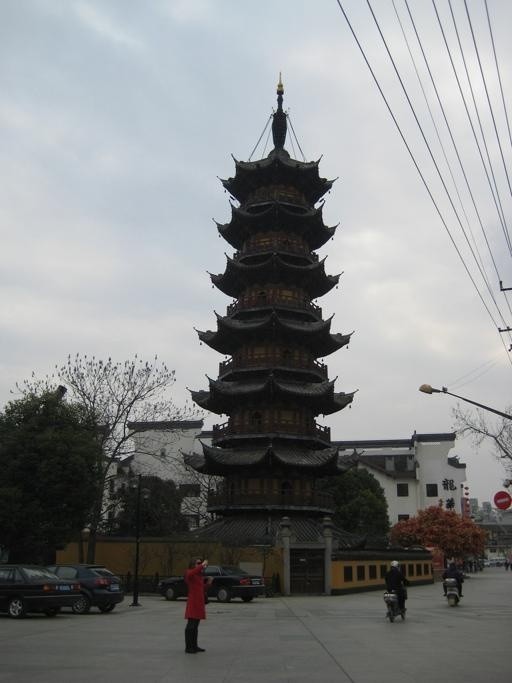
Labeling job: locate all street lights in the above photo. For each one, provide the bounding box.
[419,384,512,420]
[130,474,149,606]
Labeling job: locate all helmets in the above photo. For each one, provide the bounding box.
[391,561,398,568]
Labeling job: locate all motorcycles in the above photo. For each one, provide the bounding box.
[384,591,407,622]
[444,578,460,607]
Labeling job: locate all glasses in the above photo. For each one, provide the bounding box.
[197,562,202,564]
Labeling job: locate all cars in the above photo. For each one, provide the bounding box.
[46,564,124,614]
[159,564,265,603]
[0,565,82,618]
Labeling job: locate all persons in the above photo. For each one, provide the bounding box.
[385,561,409,611]
[184,557,214,653]
[442,563,464,597]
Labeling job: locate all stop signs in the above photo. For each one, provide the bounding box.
[494,491,512,510]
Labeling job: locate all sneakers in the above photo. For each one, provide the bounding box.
[186,646,205,653]
